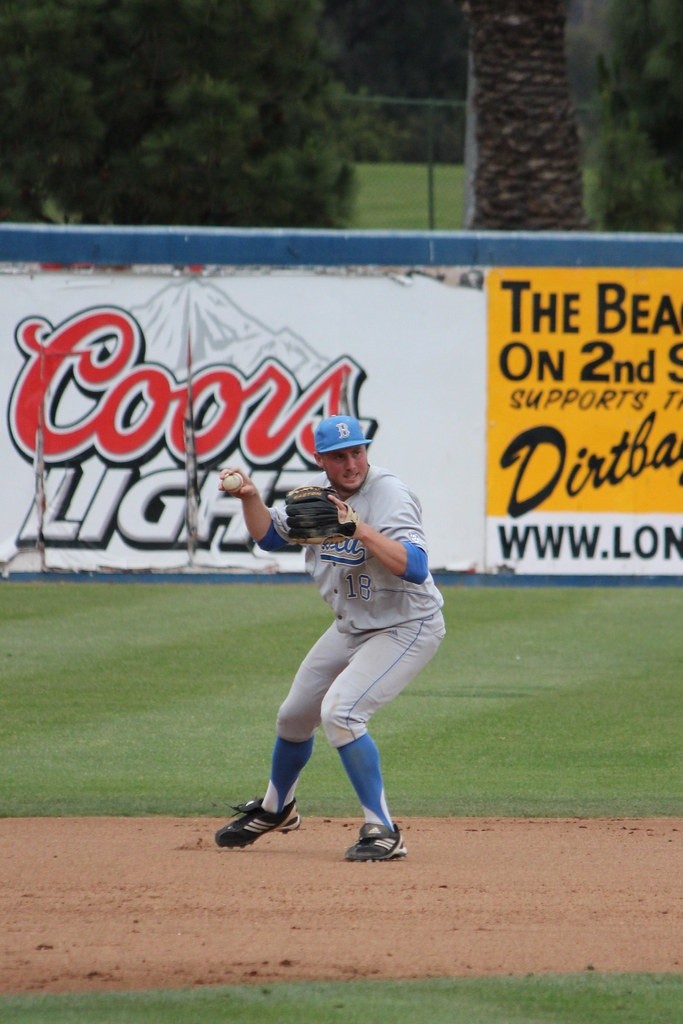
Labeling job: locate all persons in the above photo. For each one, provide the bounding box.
[215,416,446,862]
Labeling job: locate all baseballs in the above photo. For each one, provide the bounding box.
[222,471,244,494]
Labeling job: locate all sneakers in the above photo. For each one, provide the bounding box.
[345,823,408,862]
[215,798,301,849]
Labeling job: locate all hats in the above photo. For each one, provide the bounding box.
[314,415,372,453]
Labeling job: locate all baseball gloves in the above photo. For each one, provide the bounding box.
[285,486,358,545]
